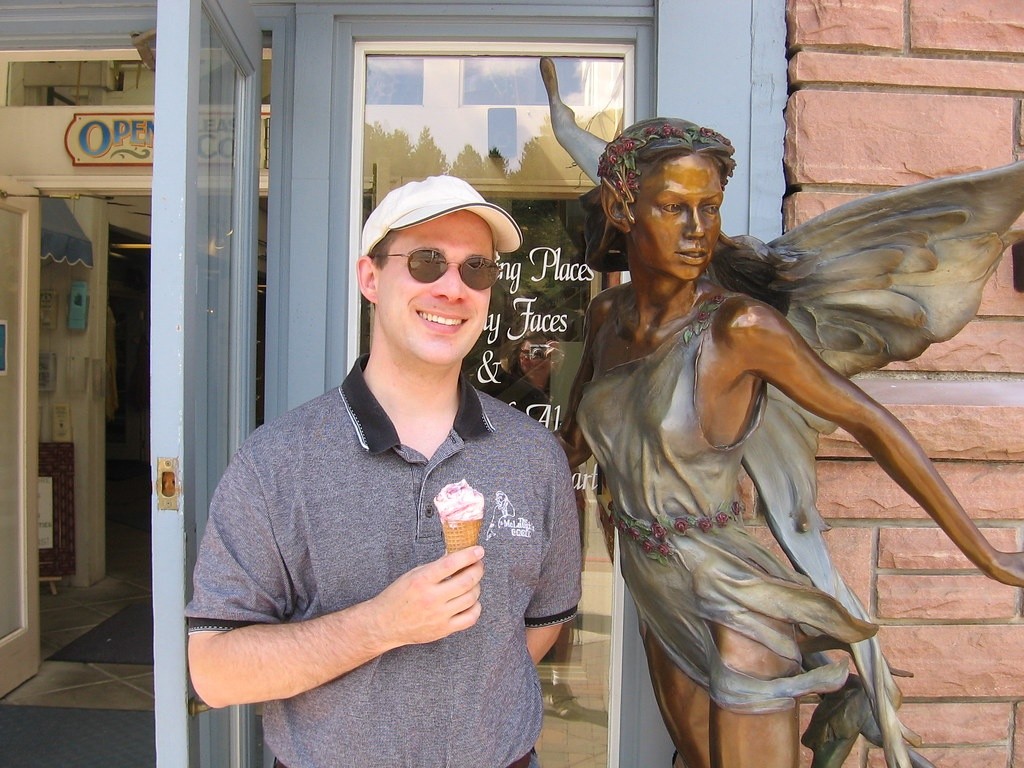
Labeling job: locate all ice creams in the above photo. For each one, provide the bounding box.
[433,478,484,576]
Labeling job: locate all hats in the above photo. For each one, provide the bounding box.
[360,175,524,257]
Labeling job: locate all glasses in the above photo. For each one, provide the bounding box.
[374,246,503,292]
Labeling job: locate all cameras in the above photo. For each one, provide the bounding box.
[530,344,552,361]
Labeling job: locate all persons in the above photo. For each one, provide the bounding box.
[184,175,581,768]
[538,52,1024,768]
[463,279,588,575]
